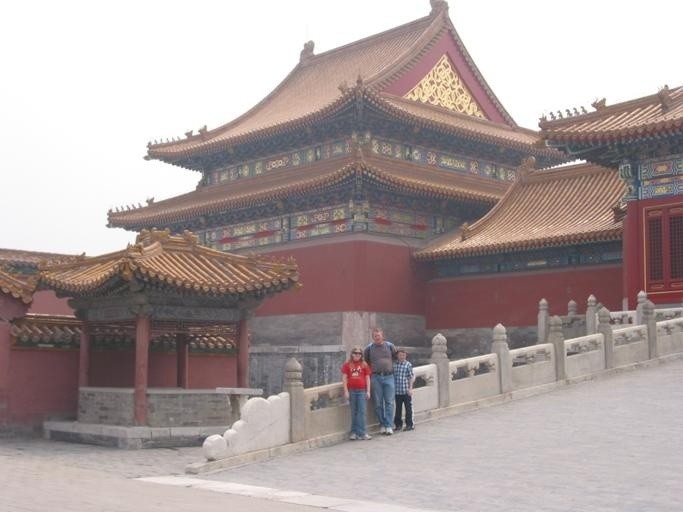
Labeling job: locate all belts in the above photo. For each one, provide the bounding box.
[371,371,393,375]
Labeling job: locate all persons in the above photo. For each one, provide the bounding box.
[363,327,399,435]
[340,346,372,439]
[392,347,416,431]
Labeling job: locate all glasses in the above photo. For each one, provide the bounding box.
[353,351,362,355]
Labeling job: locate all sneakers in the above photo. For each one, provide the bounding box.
[403,426,413,431]
[394,425,401,430]
[349,432,356,439]
[357,434,372,439]
[379,427,393,435]
